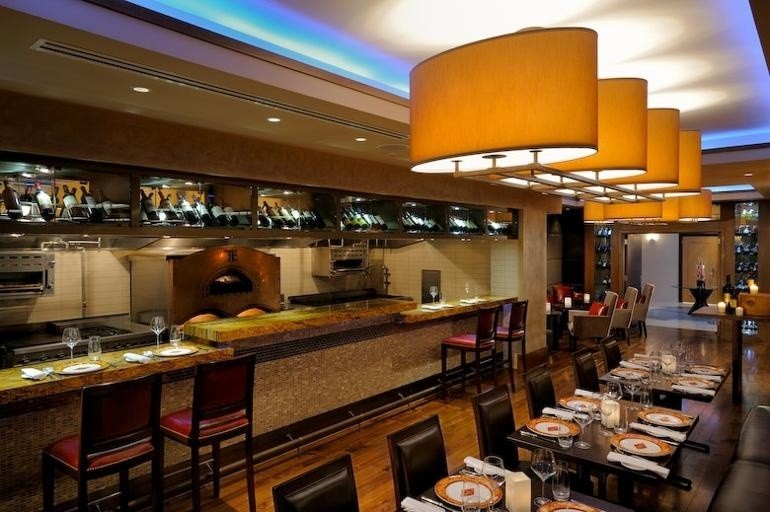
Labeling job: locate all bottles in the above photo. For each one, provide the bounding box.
[600,273,613,292]
[257,206,271,228]
[140,192,155,221]
[49,187,59,208]
[364,206,381,228]
[736,236,759,262]
[219,199,239,227]
[62,184,83,220]
[343,206,361,230]
[303,208,318,230]
[729,287,737,314]
[80,185,103,223]
[597,241,612,255]
[192,196,213,227]
[288,205,306,228]
[35,182,55,220]
[262,201,286,229]
[98,187,121,224]
[735,260,759,273]
[159,191,179,224]
[206,196,228,226]
[70,187,77,196]
[485,217,514,234]
[349,207,369,229]
[341,211,354,230]
[275,201,296,229]
[3,180,23,219]
[723,273,733,310]
[735,274,759,294]
[448,214,480,231]
[140,189,160,224]
[20,185,33,204]
[597,227,603,238]
[741,319,759,336]
[602,226,609,238]
[400,209,443,232]
[158,194,172,213]
[595,256,611,270]
[307,205,325,230]
[176,192,199,227]
[355,207,375,228]
[370,208,389,230]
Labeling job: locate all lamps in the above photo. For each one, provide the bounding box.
[533,109,678,193]
[584,189,713,224]
[410,27,598,178]
[581,130,701,204]
[493,77,648,183]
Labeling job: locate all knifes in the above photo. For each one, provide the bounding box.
[520,430,556,444]
[421,495,457,512]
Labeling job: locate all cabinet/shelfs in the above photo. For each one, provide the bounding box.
[0,341,234,512]
[591,224,611,295]
[0,152,522,240]
[738,294,770,317]
[735,200,758,289]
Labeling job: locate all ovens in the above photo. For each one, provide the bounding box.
[125,238,282,328]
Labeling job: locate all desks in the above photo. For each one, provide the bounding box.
[546,309,562,346]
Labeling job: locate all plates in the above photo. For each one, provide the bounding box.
[421,304,453,309]
[610,433,673,457]
[460,298,484,304]
[686,364,726,376]
[610,367,650,380]
[150,347,200,358]
[472,289,477,301]
[434,475,504,509]
[536,501,603,512]
[52,363,110,375]
[672,375,716,389]
[525,416,581,437]
[626,356,658,370]
[636,406,691,427]
[558,395,602,413]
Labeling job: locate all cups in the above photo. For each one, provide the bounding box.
[88,335,102,363]
[169,325,183,348]
[556,414,573,447]
[480,455,507,512]
[605,380,624,402]
[461,475,482,511]
[438,292,446,305]
[61,327,82,368]
[599,415,616,453]
[648,360,662,382]
[530,447,558,509]
[574,403,594,450]
[613,406,629,436]
[551,458,572,502]
[623,372,642,411]
[428,286,439,306]
[638,384,654,410]
[464,282,471,301]
[149,316,166,353]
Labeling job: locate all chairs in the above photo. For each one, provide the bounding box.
[600,339,623,376]
[470,385,520,463]
[386,414,450,510]
[553,285,570,294]
[733,404,770,460]
[611,286,638,347]
[631,283,655,338]
[491,299,528,394]
[41,370,164,512]
[704,461,770,512]
[441,305,498,401]
[270,452,360,512]
[566,291,619,350]
[524,366,556,423]
[162,352,257,512]
[571,347,600,393]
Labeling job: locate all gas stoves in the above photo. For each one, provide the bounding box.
[1,315,169,370]
[287,286,378,309]
